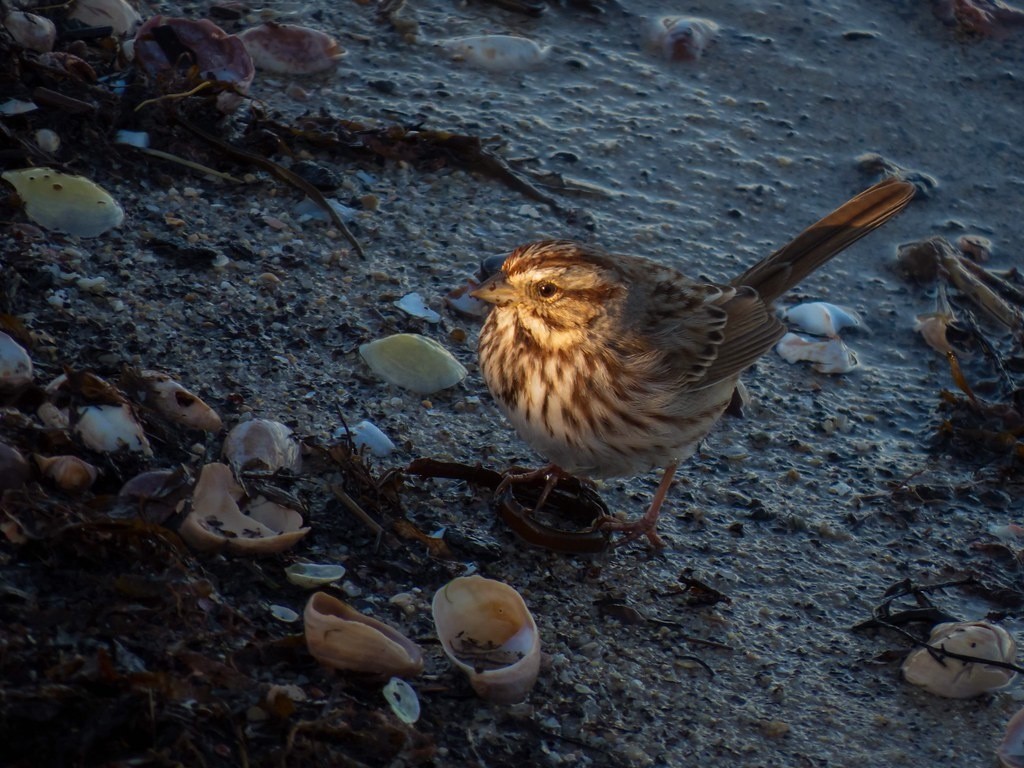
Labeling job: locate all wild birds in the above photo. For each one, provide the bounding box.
[467,177,918,552]
